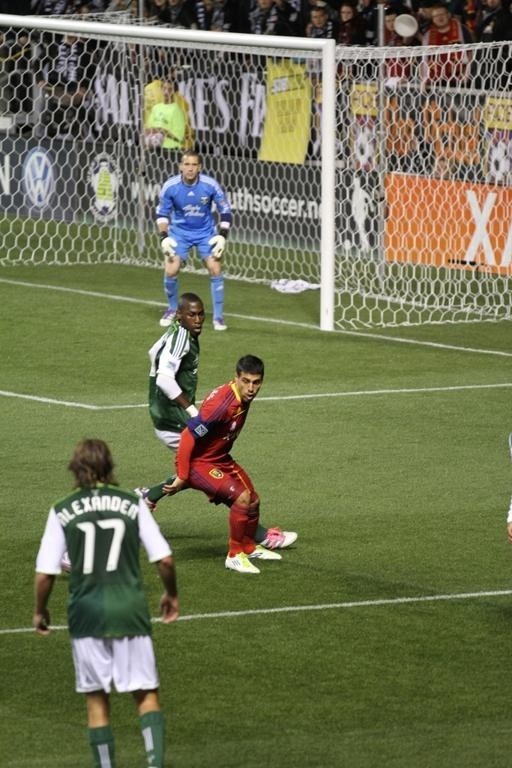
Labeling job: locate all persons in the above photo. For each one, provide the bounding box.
[145,77,186,153]
[507,499,512,545]
[32,438,180,768]
[156,154,234,331]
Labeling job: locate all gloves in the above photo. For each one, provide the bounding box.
[158,231,178,257]
[208,229,228,259]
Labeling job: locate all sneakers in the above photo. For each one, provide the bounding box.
[212,318,227,331]
[224,527,298,574]
[134,486,157,514]
[159,308,177,327]
